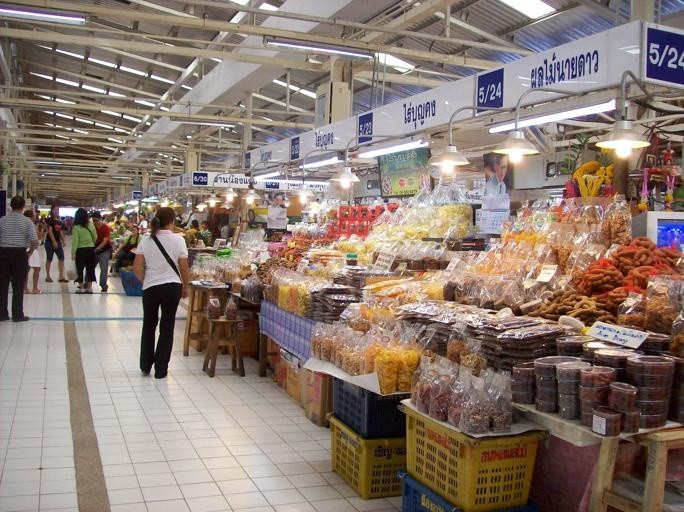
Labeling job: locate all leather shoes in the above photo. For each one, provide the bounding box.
[44,278,53,282]
[0,315,10,321]
[59,279,68,282]
[12,316,30,322]
[75,282,108,294]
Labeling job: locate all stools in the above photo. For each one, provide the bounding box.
[586,421,684,512]
[180,279,235,360]
[198,311,249,379]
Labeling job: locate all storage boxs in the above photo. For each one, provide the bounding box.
[231,311,333,430]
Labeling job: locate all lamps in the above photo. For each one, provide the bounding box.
[167,116,237,130]
[0,5,92,26]
[490,86,584,164]
[589,70,657,162]
[480,86,630,136]
[424,103,514,174]
[195,132,441,214]
[370,50,417,75]
[259,33,374,59]
[497,0,558,23]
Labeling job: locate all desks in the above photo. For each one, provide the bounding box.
[501,396,682,511]
[257,295,335,426]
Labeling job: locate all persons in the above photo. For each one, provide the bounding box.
[0,194,38,322]
[248,210,255,221]
[131,207,192,378]
[485,154,508,195]
[36,205,211,295]
[218,222,229,238]
[269,193,283,209]
[20,209,42,294]
